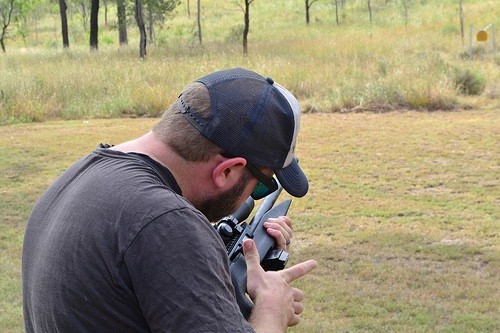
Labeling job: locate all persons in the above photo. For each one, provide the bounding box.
[22,68,320,333]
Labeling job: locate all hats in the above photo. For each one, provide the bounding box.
[177,67,308,198]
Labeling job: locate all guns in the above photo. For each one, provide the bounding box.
[211,156,299,322]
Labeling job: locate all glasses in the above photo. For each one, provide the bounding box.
[218,152,278,200]
